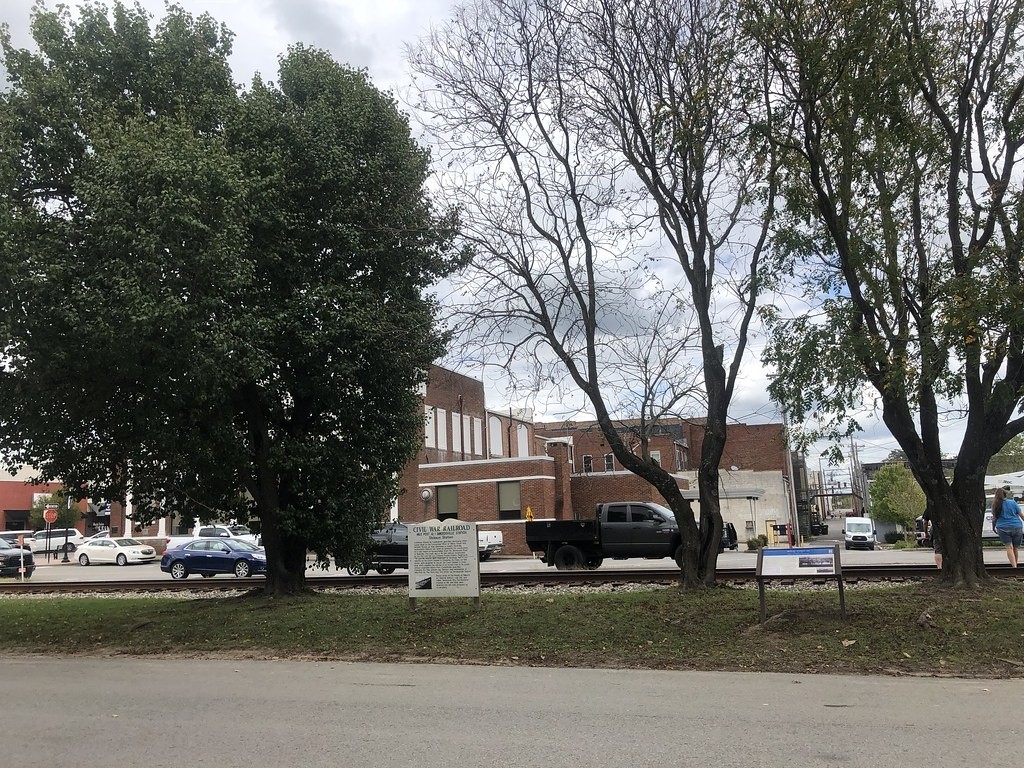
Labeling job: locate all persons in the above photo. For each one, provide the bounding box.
[992,488,1024,568]
[923,507,943,568]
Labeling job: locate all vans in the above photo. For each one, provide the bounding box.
[839,516,878,551]
[0,529,36,543]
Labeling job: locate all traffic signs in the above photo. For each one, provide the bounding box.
[44,503,59,510]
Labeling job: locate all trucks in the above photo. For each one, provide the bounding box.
[914,495,1024,549]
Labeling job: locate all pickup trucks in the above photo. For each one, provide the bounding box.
[524,500,739,570]
[162,523,260,551]
[478,528,507,562]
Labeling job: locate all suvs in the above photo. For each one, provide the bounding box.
[0,534,32,552]
[23,528,84,554]
[333,520,421,575]
[0,537,36,579]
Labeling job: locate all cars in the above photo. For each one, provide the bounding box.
[74,537,157,566]
[84,529,110,543]
[160,536,267,580]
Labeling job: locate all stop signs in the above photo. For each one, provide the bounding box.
[45,508,58,523]
